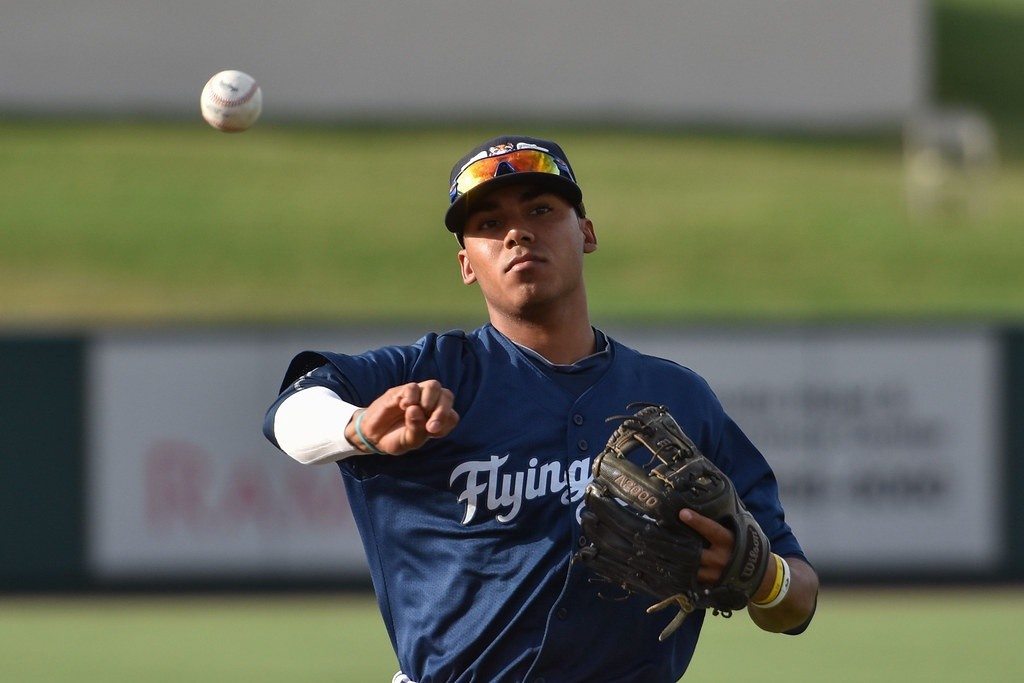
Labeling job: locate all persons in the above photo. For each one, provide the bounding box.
[261,137,824,683]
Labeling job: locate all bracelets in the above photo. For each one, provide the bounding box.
[355,407,388,457]
[747,550,792,609]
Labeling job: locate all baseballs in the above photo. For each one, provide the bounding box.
[200,70,262,132]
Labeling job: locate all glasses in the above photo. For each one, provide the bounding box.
[449,149,575,202]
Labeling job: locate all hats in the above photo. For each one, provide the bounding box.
[444,134,582,250]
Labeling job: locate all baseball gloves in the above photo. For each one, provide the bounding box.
[580,404,772,612]
